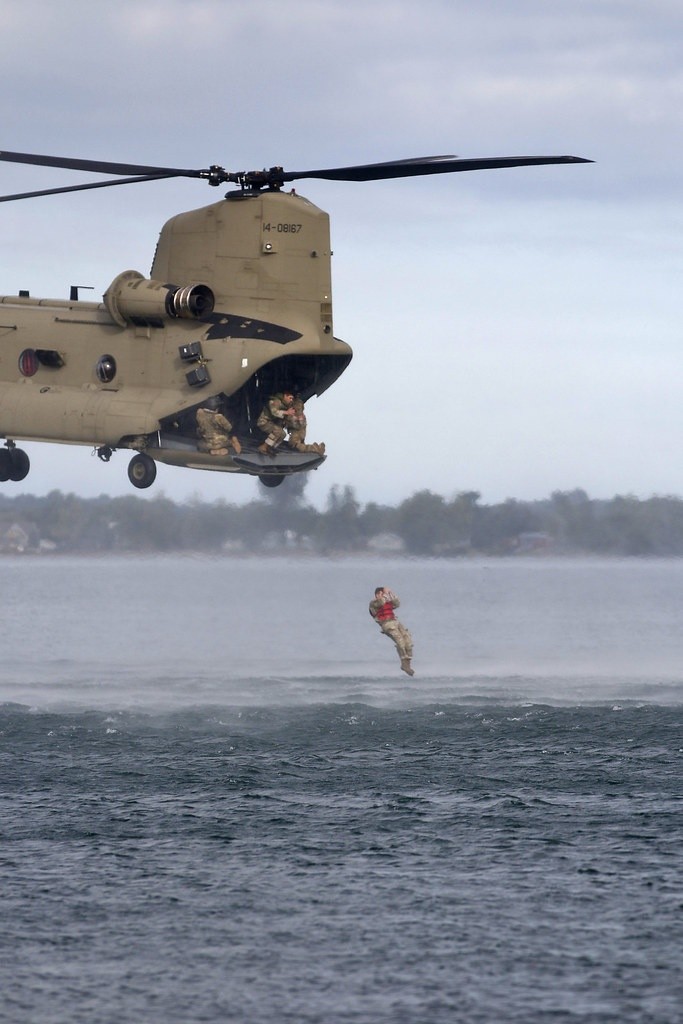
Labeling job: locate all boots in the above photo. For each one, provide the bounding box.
[401,658,414,676]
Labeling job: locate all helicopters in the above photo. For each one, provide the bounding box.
[0,151,596,489]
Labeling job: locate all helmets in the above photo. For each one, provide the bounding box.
[205,397,224,411]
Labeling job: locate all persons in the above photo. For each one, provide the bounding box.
[369,586,414,677]
[255,389,326,457]
[195,396,242,455]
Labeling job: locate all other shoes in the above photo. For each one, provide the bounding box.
[230,436,241,455]
[210,448,228,455]
[311,442,325,456]
[258,443,268,455]
